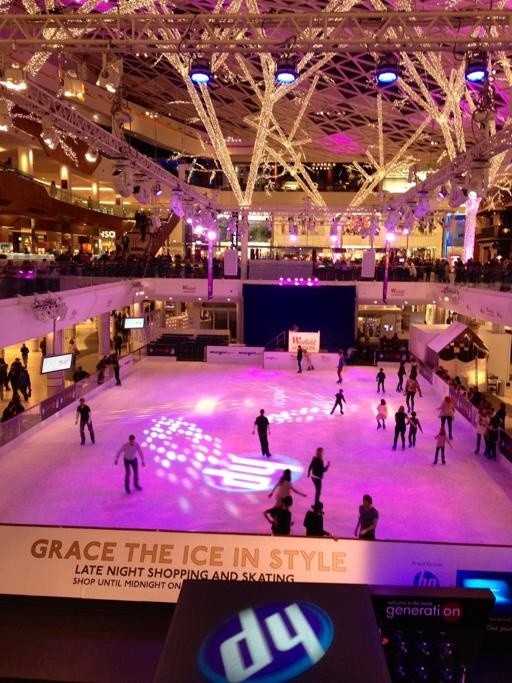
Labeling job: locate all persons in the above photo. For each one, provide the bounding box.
[327,321,506,466]
[268,469,306,510]
[0,305,153,430]
[354,494,379,540]
[304,349,314,370]
[115,435,146,494]
[251,408,272,457]
[296,345,302,373]
[302,502,337,541]
[307,448,331,505]
[263,497,285,535]
[275,496,295,536]
[74,399,96,446]
[1,243,512,294]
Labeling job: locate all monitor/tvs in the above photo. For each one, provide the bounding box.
[456,570,512,613]
[41,353,73,374]
[123,317,145,329]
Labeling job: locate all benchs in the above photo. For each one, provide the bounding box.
[147,333,230,362]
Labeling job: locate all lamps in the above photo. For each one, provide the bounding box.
[40,48,489,240]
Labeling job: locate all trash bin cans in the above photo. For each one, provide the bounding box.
[497,380,506,397]
